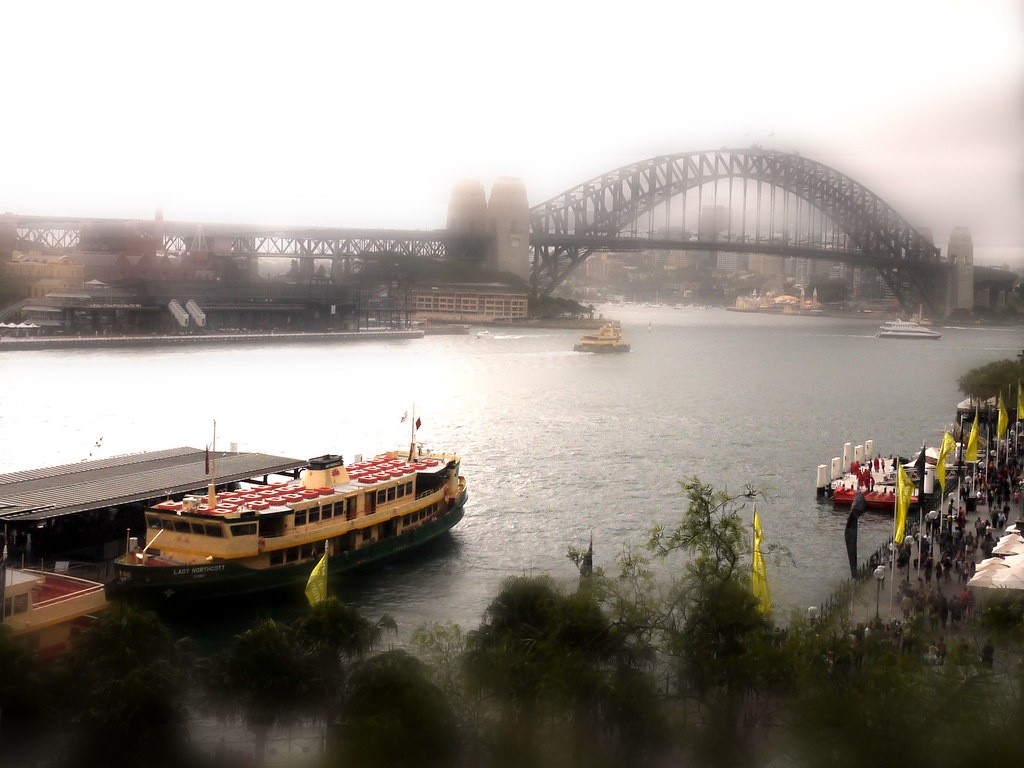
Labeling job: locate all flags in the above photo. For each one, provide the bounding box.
[937,379,1024,498]
[896,464,915,546]
[304,553,328,607]
[414,416,424,431]
[915,446,925,507]
[753,513,771,612]
[844,493,870,579]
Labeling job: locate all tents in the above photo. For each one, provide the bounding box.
[991,534,1024,555]
[1004,522,1024,536]
[966,565,1024,590]
[973,555,1024,573]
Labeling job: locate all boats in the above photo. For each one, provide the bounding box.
[0,524,112,668]
[410,320,469,335]
[475,330,494,340]
[874,316,944,340]
[831,487,928,511]
[572,320,631,354]
[110,418,471,625]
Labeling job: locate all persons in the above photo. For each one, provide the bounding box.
[850,409,1024,673]
